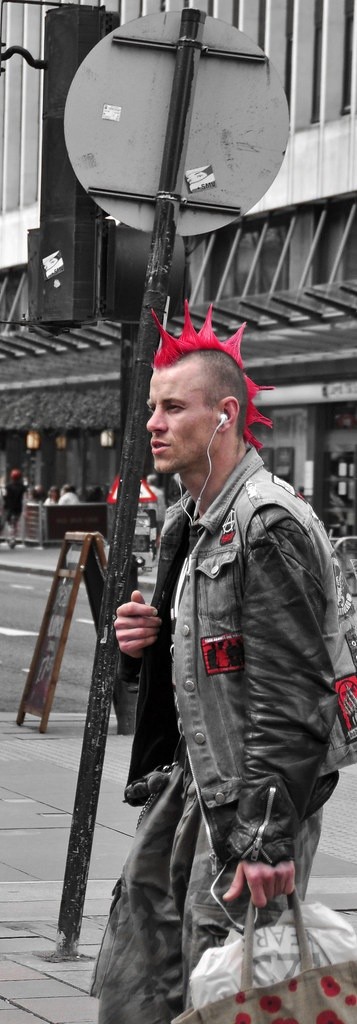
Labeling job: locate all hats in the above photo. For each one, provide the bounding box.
[10,470,21,478]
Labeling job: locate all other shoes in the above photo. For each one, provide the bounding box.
[8,539,15,549]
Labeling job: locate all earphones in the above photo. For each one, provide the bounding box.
[216,413,227,430]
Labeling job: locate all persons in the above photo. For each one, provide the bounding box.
[91,305,357,1024]
[1,469,80,549]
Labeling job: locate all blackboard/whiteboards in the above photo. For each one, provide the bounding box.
[16,531,92,718]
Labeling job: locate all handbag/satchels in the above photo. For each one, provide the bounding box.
[168,881,357,1024]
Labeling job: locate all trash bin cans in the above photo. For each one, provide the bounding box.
[26,503,40,543]
